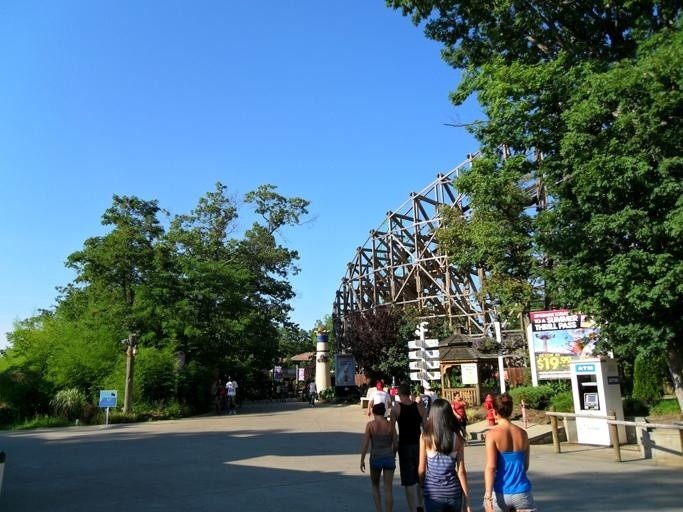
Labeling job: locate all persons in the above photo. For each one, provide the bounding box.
[449,392,468,447]
[389,383,427,512]
[413,386,431,433]
[417,399,473,512]
[359,403,398,512]
[479,392,538,512]
[224,375,239,414]
[367,382,391,420]
[307,380,317,408]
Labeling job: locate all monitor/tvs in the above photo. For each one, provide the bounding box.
[584,394,598,405]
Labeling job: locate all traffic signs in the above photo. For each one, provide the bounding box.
[408,338,441,390]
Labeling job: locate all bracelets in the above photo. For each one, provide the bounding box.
[483,496,492,502]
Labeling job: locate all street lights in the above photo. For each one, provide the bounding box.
[489,310,505,394]
[124,334,138,413]
